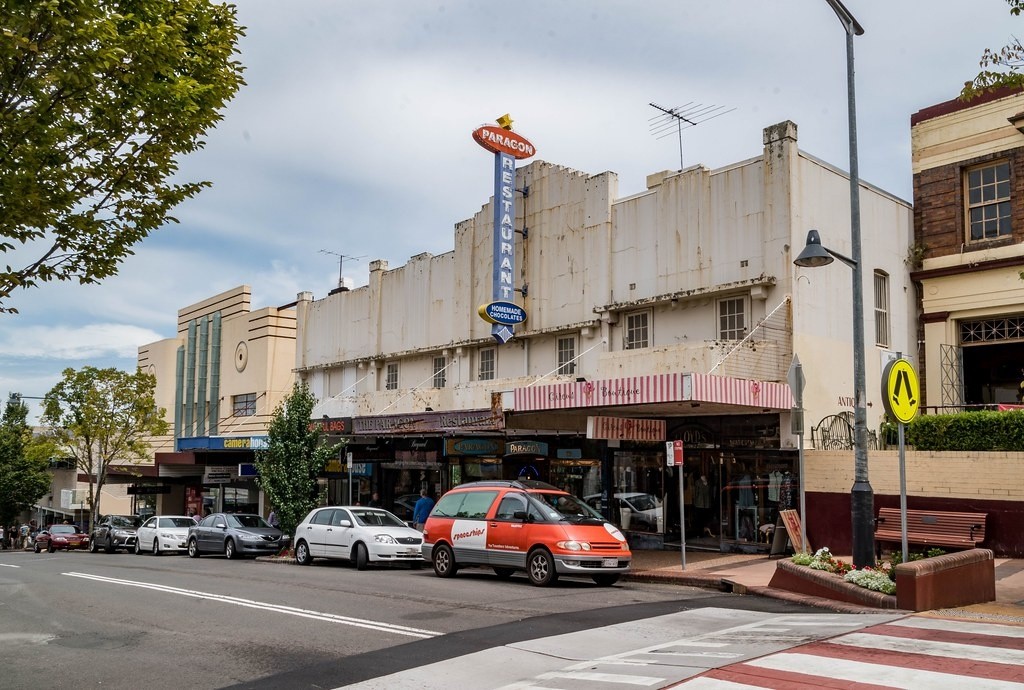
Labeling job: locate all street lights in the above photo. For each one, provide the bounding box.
[792,0,878,569]
[4,393,102,529]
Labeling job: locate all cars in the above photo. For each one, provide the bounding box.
[293,506,426,571]
[573,493,663,534]
[33,524,90,553]
[185,512,291,560]
[134,514,199,556]
[89,514,147,554]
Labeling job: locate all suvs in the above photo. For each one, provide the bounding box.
[419,479,632,587]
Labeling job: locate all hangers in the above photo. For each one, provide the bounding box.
[784,469,791,475]
[773,467,779,472]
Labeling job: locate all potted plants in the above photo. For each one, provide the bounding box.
[903,242,932,271]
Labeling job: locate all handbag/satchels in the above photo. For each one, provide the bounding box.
[28,535,31,543]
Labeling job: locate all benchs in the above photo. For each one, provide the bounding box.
[874,507,989,560]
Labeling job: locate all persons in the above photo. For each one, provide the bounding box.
[268,510,282,531]
[353,502,361,506]
[623,466,634,493]
[189,510,202,522]
[413,489,435,532]
[693,475,711,539]
[367,493,382,508]
[0,523,30,550]
[202,507,213,518]
